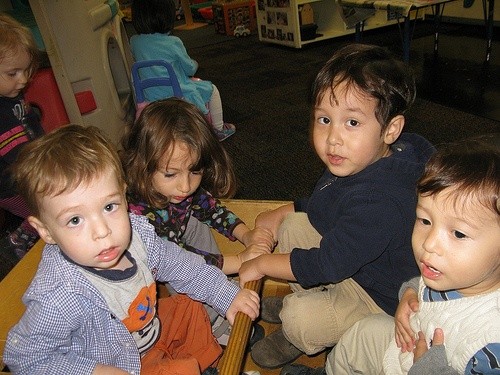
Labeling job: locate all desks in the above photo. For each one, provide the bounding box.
[335,0,456,67]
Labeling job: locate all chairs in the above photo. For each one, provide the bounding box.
[131,59,211,125]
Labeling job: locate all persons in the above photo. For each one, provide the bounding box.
[3,125,261,375]
[238,43,437,368]
[281,133,500,375]
[128,0,236,142]
[0,13,51,264]
[118,97,275,352]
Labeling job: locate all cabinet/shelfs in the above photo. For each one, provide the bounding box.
[255,0,425,49]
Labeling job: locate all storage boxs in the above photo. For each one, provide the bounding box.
[211,2,256,36]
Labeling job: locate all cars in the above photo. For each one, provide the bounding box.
[234,24,251,36]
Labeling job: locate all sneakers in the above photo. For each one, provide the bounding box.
[212,123,236,142]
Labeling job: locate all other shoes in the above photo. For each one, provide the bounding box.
[211,315,265,352]
[250,325,304,369]
[259,297,286,324]
[280,363,327,375]
